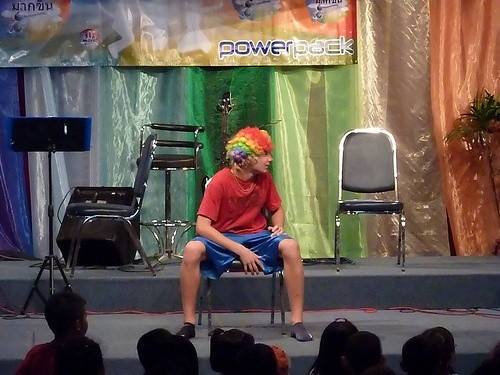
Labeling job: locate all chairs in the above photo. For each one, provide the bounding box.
[198,175,286,336]
[335,128,407,273]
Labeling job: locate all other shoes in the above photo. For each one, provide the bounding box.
[288,321,313,342]
[176,321,196,339]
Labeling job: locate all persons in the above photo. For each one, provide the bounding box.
[13,287,106,375]
[174,126,314,342]
[135,316,500,375]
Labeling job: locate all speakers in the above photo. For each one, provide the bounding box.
[56,186,140,267]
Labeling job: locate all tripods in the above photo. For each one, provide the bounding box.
[11,117,91,319]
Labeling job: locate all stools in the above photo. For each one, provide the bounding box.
[135,123,206,271]
